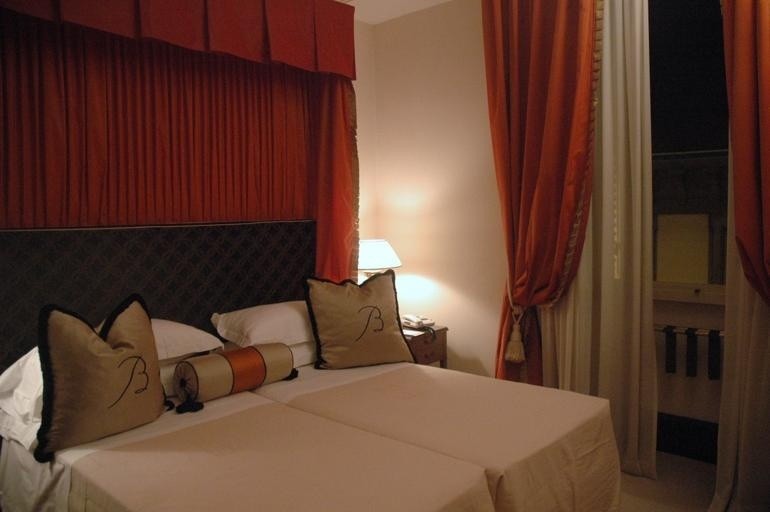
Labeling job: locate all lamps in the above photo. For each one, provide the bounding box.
[359,238,401,279]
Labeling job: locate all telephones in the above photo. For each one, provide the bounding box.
[402,314,435,329]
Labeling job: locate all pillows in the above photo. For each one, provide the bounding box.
[222,341,318,370]
[32,293,175,462]
[302,269,417,369]
[211,300,315,348]
[0,360,182,453]
[0,317,225,430]
[174,342,299,414]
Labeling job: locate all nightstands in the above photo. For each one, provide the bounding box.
[402,324,449,369]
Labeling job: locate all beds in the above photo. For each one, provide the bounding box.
[0,219,621,512]
